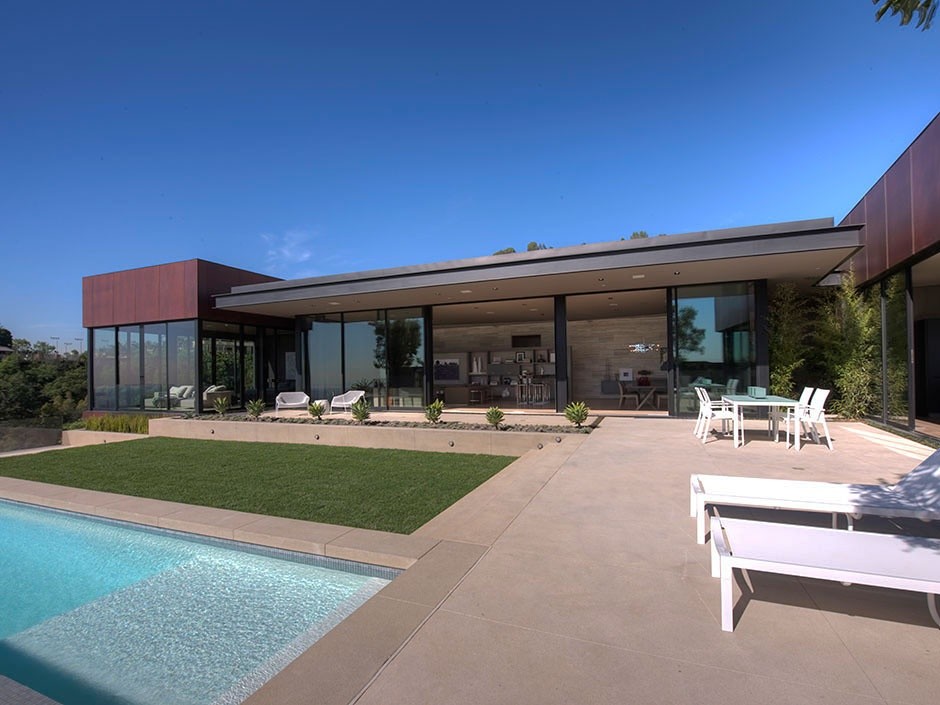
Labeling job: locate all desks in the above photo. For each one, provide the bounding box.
[630,384,664,410]
[313,399,330,415]
[688,383,725,411]
[720,394,802,451]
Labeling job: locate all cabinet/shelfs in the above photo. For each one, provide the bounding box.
[465,346,571,407]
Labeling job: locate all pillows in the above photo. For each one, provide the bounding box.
[153,384,226,400]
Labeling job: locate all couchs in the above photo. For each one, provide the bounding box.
[143,385,195,408]
[180,390,232,411]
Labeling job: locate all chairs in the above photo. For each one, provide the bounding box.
[693,386,834,451]
[690,448,940,544]
[275,391,310,414]
[674,378,739,413]
[330,389,365,417]
[617,379,670,410]
[709,515,940,633]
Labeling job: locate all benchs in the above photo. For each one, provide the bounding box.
[599,379,668,399]
